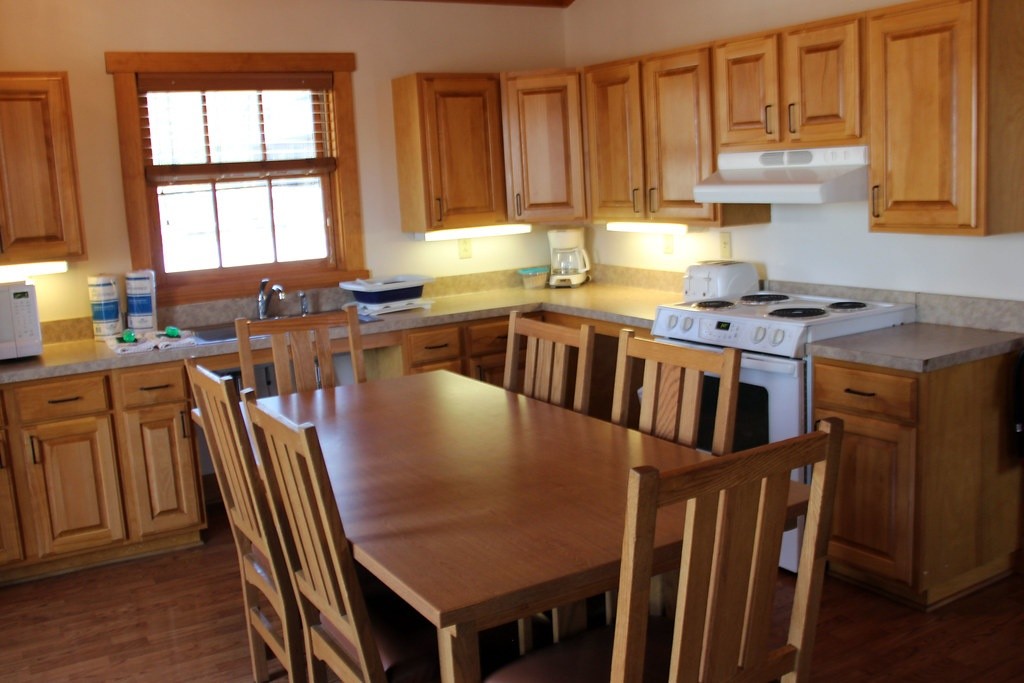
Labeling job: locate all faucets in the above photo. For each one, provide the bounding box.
[257,278,287,320]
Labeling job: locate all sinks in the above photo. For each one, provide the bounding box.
[191,323,265,344]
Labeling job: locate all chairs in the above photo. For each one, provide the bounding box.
[184,302,845,683]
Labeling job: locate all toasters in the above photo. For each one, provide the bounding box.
[685,260,759,301]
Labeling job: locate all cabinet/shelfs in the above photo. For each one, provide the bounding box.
[0,376,130,569]
[504,72,582,226]
[710,16,862,150]
[867,0,985,232]
[806,369,919,584]
[584,48,714,220]
[0,71,86,268]
[111,369,205,542]
[393,67,505,234]
[407,316,547,389]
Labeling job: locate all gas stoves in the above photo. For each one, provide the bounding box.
[650,289,916,358]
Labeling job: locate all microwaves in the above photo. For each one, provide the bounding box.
[0,281,42,358]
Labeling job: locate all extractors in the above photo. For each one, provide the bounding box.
[693,146,869,203]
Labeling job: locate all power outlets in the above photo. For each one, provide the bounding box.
[720,233,733,258]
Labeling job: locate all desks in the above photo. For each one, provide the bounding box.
[191,366,812,683]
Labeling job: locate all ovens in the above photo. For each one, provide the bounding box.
[637,338,812,572]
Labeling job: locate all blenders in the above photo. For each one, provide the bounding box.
[547,229,590,288]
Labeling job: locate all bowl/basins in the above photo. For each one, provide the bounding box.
[517,268,549,289]
[340,273,431,304]
